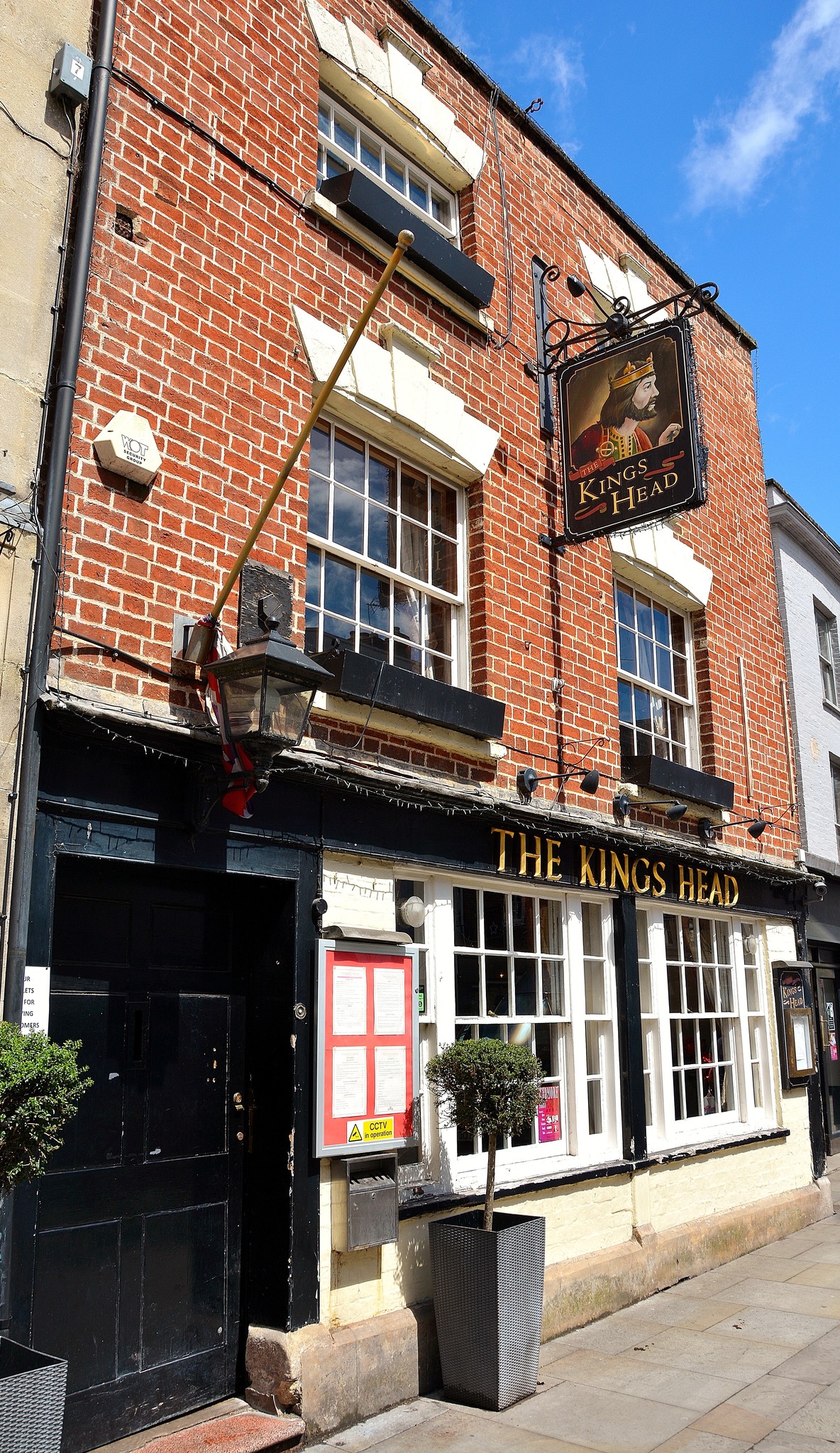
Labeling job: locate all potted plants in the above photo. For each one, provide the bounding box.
[0,1019,95,1453]
[420,1030,547,1412]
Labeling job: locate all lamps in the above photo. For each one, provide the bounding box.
[181,614,339,845]
[611,792,688,823]
[516,767,599,796]
[698,816,767,840]
